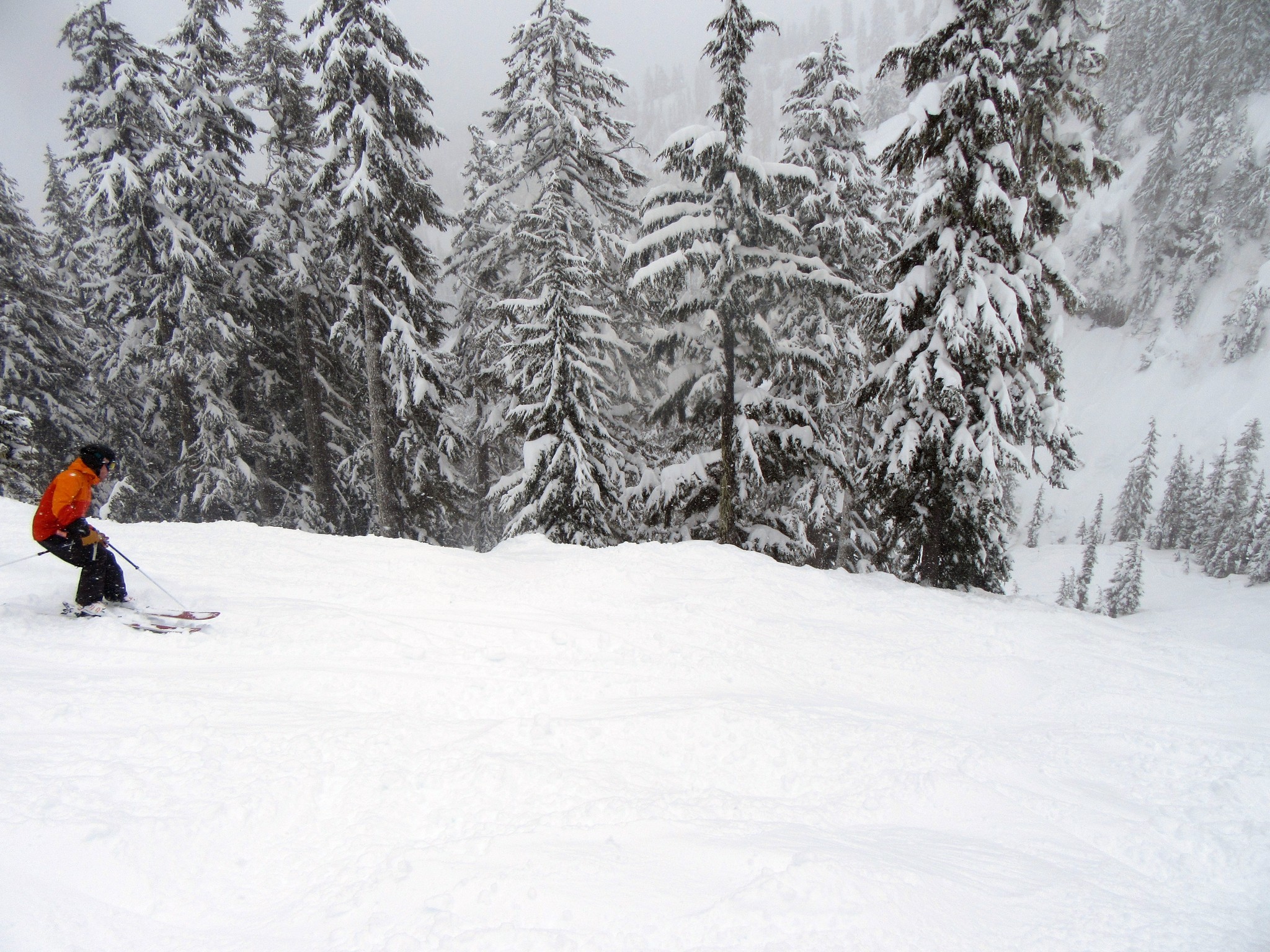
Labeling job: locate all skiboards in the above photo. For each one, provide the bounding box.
[56,599,220,634]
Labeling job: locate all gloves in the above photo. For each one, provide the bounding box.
[89,525,110,547]
[77,529,99,545]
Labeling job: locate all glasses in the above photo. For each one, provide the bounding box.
[103,460,115,471]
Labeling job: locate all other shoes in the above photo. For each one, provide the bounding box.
[77,600,108,616]
[106,595,139,610]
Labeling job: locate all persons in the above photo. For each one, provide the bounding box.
[32,443,142,617]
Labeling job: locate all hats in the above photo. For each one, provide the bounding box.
[80,442,116,477]
